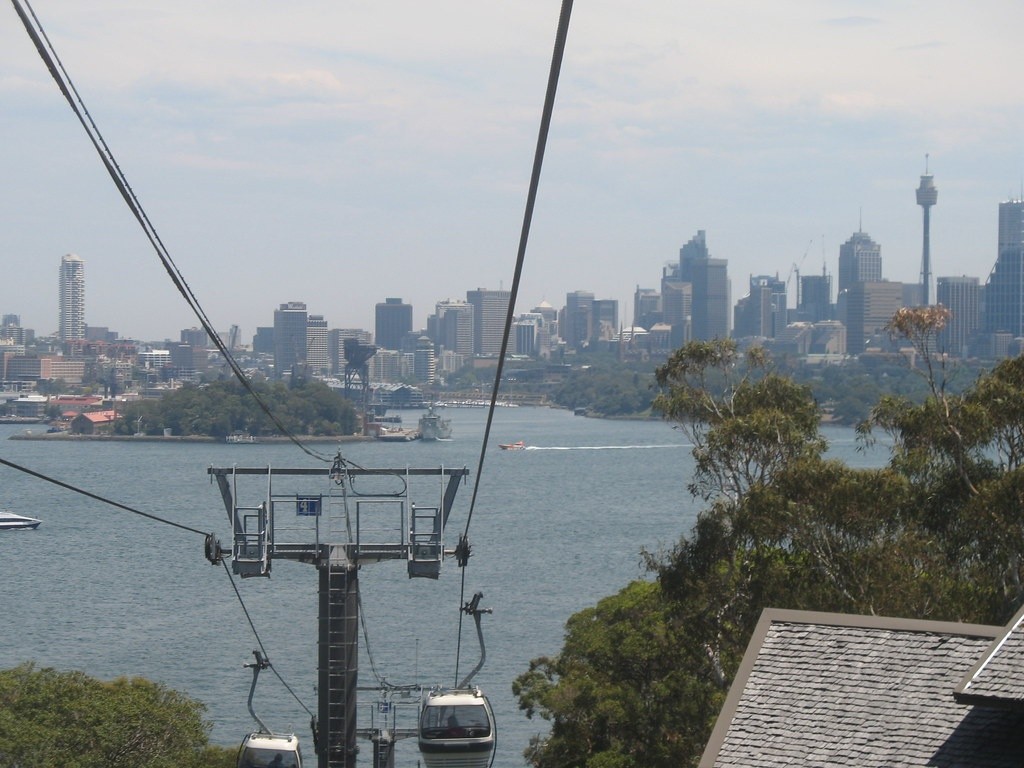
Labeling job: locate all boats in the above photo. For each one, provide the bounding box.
[0,512,42,529]
[498,442,526,450]
[418,406,453,439]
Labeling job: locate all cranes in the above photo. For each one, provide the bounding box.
[788,238,813,309]
[821,235,827,277]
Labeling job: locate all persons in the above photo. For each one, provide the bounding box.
[270,753,282,768]
[447,716,467,737]
